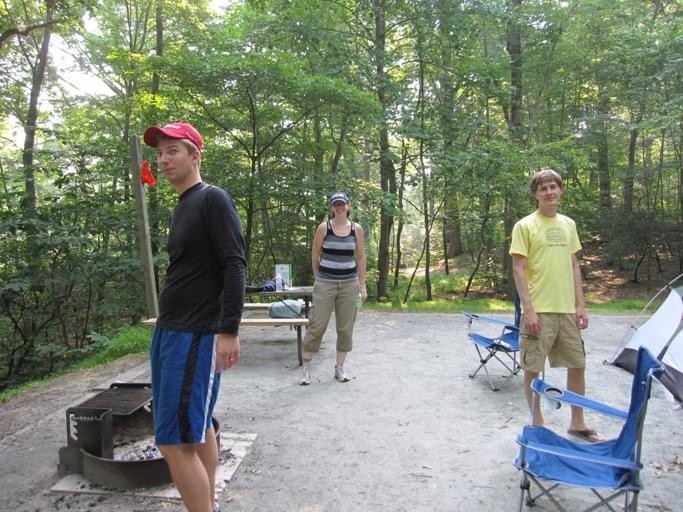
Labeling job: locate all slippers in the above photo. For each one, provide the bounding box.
[567,428,605,442]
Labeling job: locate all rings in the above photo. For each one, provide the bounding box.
[229,357,234,361]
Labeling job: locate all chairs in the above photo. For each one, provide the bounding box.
[513,345,664,512]
[462,290,521,392]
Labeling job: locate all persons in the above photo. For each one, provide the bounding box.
[145,121,247,512]
[298,193,368,385]
[508,169,602,444]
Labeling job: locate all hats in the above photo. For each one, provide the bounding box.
[143,122,203,152]
[331,192,349,206]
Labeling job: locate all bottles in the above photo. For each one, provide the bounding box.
[276,272,282,292]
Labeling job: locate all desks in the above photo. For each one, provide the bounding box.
[245,286,314,366]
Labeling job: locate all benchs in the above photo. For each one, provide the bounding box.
[142,317,311,365]
[244,303,314,311]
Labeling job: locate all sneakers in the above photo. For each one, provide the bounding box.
[334,365,351,382]
[298,367,312,385]
